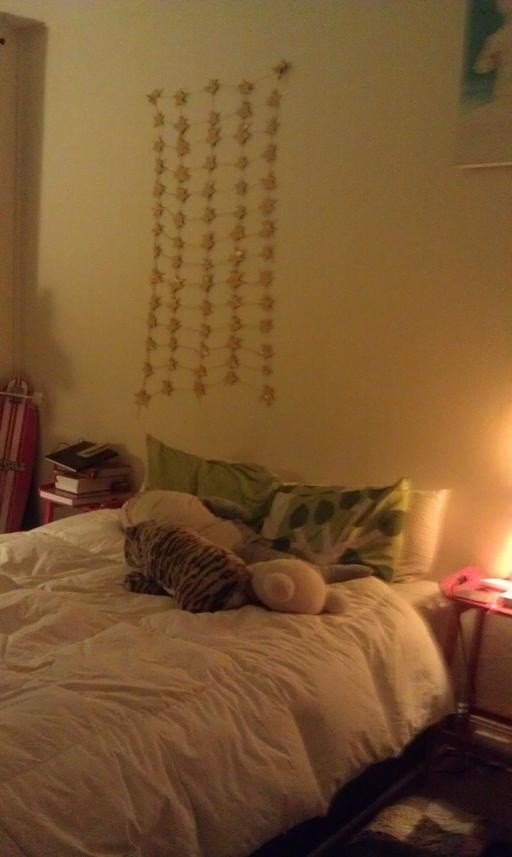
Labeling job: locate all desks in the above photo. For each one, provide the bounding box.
[438,564,512,757]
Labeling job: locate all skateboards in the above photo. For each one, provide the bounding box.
[0,377,44,535]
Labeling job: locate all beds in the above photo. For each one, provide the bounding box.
[1,506,448,856]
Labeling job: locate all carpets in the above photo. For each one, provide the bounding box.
[311,739,512,856]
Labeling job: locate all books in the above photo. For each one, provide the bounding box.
[37,437,133,508]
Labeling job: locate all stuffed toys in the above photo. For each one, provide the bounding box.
[123,520,254,614]
[232,533,374,616]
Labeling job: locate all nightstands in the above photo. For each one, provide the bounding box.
[38,482,130,523]
